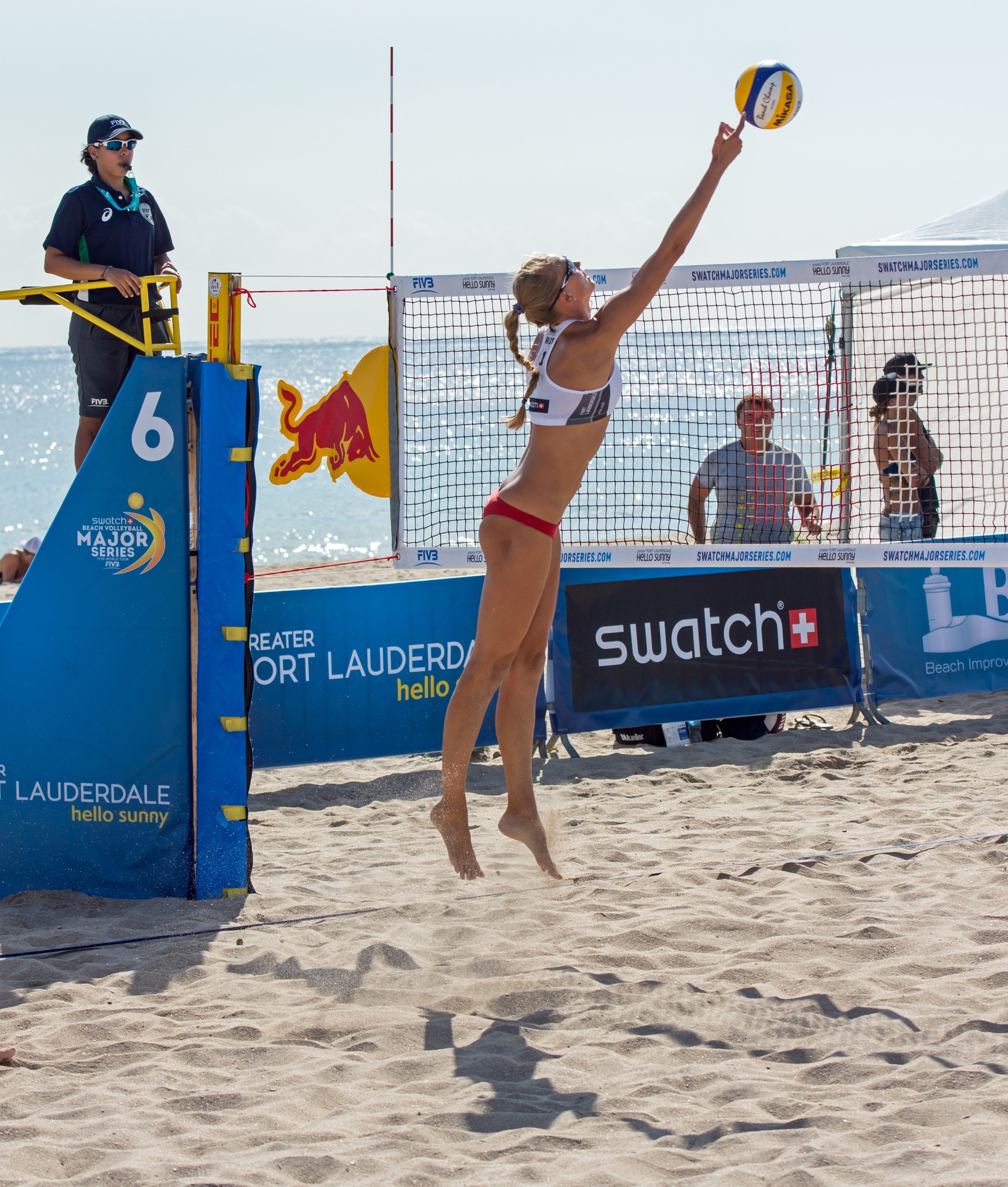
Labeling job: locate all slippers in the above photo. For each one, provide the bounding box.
[795,714,834,730]
[788,720,822,730]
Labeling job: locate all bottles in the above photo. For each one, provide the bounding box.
[688,720,702,744]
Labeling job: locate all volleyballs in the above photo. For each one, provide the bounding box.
[734,60,803,131]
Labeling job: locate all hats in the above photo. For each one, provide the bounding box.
[872,373,923,403]
[884,353,933,375]
[87,114,144,145]
[25,536,42,554]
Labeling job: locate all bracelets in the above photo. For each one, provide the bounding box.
[925,468,929,479]
[101,265,113,280]
[808,515,820,522]
[158,262,178,276]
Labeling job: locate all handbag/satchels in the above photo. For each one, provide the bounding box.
[612,724,667,747]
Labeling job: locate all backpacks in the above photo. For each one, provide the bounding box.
[685,719,721,742]
[716,713,787,740]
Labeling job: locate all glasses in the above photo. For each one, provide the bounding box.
[548,256,578,313]
[87,139,137,151]
[915,370,923,379]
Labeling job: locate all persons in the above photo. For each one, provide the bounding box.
[882,352,944,539]
[0,537,43,586]
[870,373,930,543]
[43,114,181,473]
[429,111,746,880]
[688,392,822,545]
